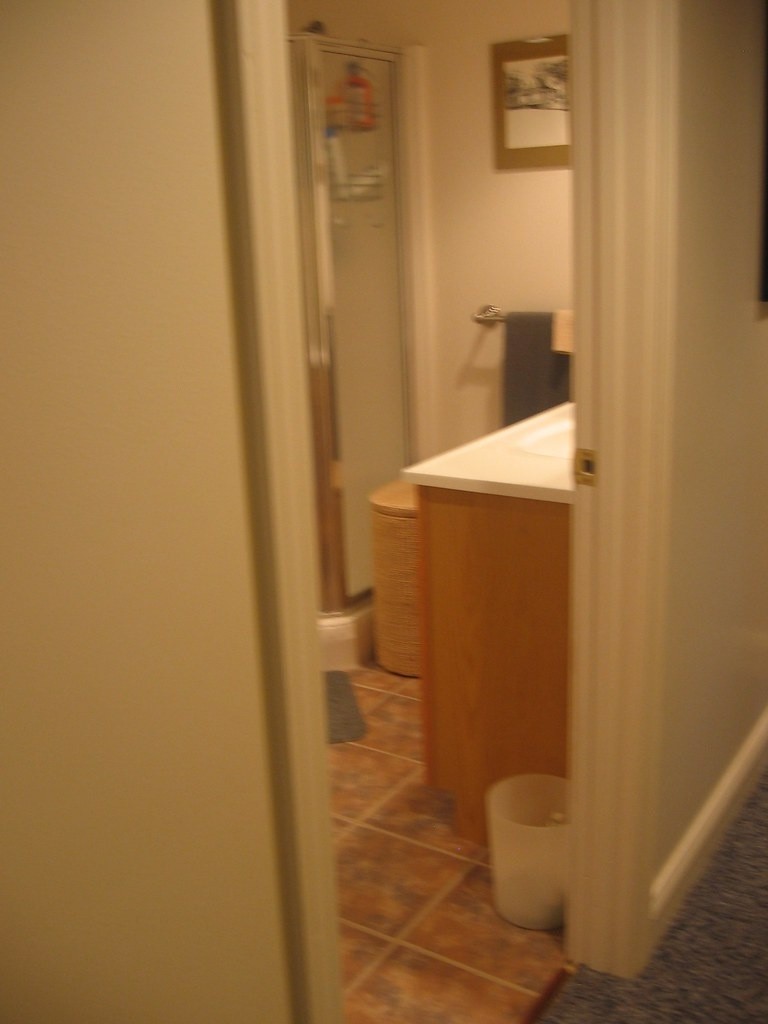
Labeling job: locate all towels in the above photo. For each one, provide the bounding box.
[503,309,575,425]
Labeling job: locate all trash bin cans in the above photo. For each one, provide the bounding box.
[485,773,570,932]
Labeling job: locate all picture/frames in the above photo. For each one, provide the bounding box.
[487,32,579,173]
